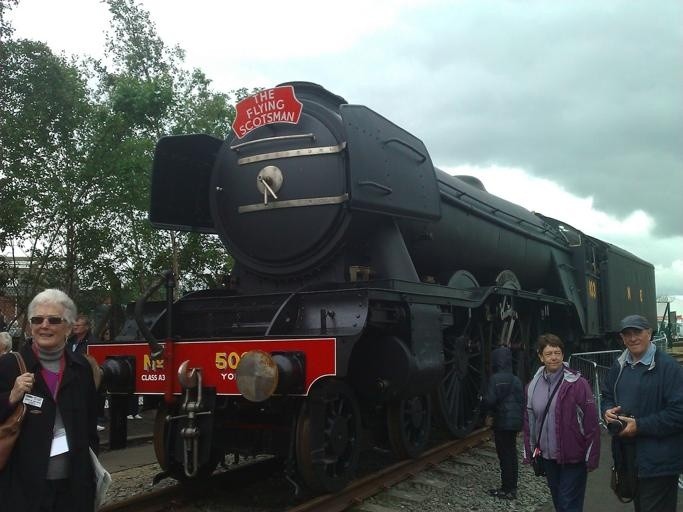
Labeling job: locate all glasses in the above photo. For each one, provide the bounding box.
[30,317,66,324]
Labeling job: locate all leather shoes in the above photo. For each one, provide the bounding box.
[487,488,516,499]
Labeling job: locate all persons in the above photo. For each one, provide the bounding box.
[0,312,143,451]
[599,315,682,512]
[0,289,100,512]
[478,347,526,500]
[659,321,683,349]
[520,333,600,512]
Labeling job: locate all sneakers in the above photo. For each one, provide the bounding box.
[97,425,105,430]
[127,414,143,419]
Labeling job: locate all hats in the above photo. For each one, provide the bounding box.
[620,314,650,332]
[0,401,27,470]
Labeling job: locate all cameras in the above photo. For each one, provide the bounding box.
[607,413,639,433]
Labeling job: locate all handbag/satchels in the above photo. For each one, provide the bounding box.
[485,416,494,426]
[610,466,637,504]
[533,444,544,477]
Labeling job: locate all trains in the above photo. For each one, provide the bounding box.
[87,81,658,496]
